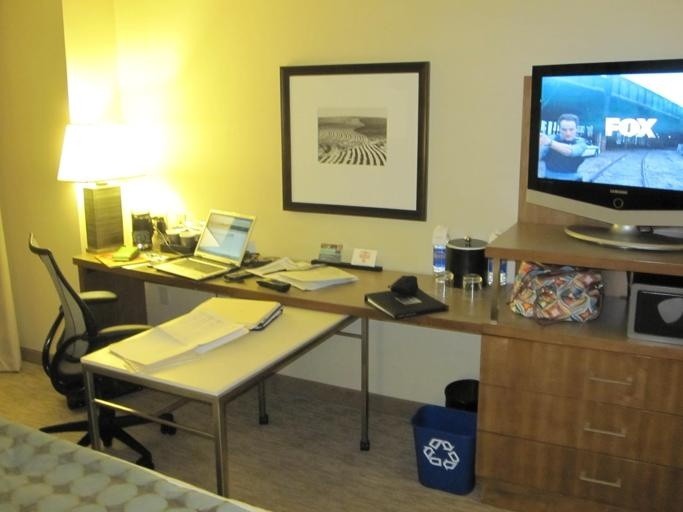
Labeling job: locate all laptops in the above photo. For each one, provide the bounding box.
[153,209,256,282]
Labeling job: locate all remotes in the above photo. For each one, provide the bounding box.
[257,278,291,293]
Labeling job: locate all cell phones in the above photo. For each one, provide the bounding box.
[224,270,254,281]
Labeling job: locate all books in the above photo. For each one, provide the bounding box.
[245,255,360,292]
[111,295,290,375]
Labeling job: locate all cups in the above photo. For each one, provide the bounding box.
[164,226,201,249]
[459,274,484,300]
[432,270,454,298]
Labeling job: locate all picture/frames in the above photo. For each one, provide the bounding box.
[280,61,429,220]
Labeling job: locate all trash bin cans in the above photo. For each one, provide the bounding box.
[411,405,476,495]
[445,379,479,413]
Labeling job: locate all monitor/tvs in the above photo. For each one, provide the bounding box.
[525,58,683,252]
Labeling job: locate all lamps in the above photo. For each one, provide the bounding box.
[57,119,147,254]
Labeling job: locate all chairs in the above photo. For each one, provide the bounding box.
[28,232,177,470]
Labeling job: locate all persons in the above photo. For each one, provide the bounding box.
[537,112,587,180]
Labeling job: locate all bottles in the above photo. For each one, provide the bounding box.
[488,258,506,287]
[432,224,448,273]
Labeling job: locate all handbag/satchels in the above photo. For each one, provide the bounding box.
[508,260,606,327]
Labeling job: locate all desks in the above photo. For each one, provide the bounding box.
[80,297,370,497]
[73,226,683,512]
